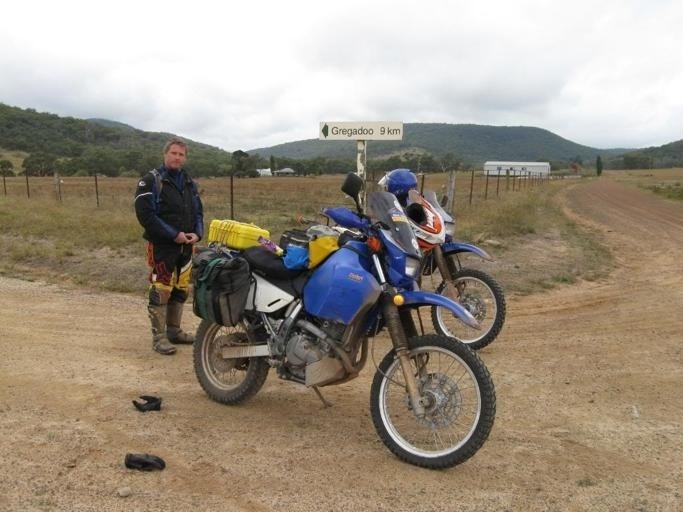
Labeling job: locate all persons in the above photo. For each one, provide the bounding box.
[135,140,204,355]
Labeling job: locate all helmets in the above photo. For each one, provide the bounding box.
[404,188,447,253]
[376,166,419,202]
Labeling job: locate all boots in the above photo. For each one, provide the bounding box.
[147,304,177,355]
[166,297,198,345]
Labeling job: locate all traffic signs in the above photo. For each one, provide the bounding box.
[316,119,403,142]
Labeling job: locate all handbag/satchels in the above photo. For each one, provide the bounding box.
[186,242,252,328]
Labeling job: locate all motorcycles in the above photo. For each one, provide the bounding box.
[280,167,507,350]
[185,170,499,471]
